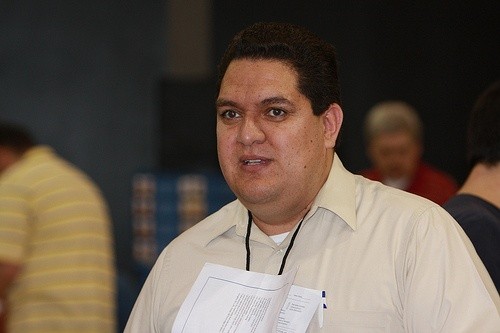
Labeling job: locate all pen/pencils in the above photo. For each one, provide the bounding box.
[322,291,328,309]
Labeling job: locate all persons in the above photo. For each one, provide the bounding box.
[124,22,500,333]
[441,82,500,296]
[362,100,458,206]
[0,125,117,333]
